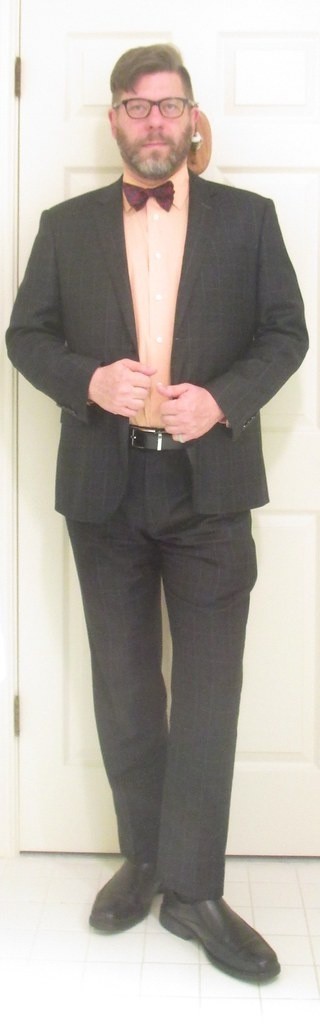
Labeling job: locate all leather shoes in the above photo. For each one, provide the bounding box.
[160,893,281,981]
[89,859,162,930]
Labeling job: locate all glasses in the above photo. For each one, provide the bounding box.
[113,98,195,119]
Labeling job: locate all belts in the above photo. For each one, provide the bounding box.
[129,425,185,451]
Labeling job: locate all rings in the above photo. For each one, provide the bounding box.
[178,434,185,444]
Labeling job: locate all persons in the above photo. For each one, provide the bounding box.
[6,44,309,980]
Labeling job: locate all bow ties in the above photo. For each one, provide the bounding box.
[122,180,175,212]
